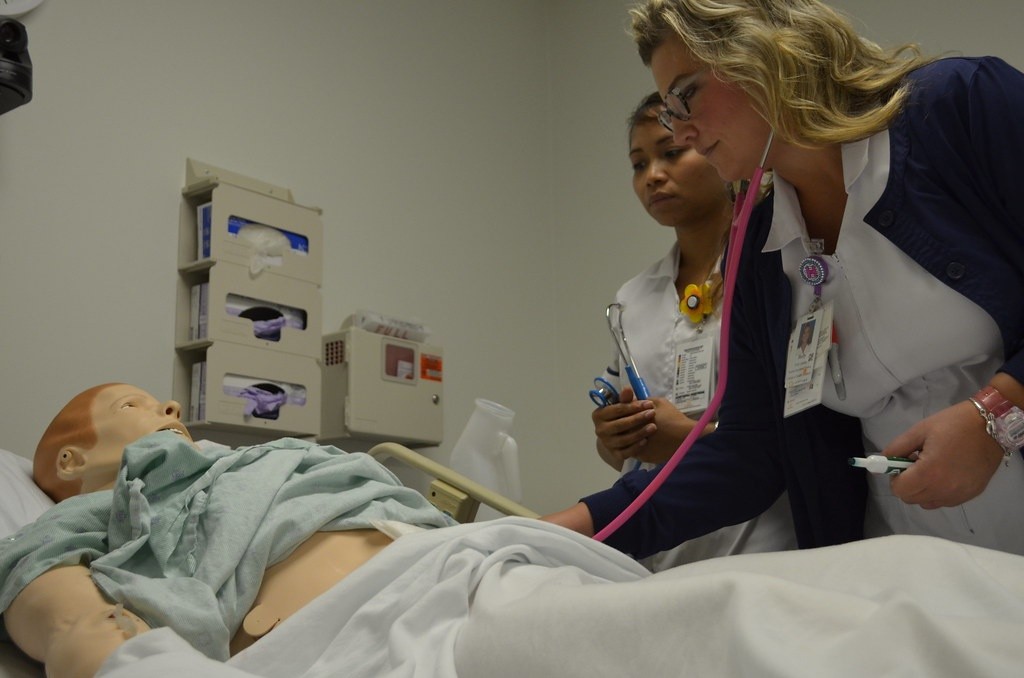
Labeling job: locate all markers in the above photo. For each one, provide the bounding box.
[848,454,913,476]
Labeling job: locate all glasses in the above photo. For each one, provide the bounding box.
[658,65,711,133]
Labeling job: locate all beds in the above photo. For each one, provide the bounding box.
[0,438,1022,676]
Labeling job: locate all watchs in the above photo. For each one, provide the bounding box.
[971,386,1024,457]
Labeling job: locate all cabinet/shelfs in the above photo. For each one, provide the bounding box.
[169,155,444,448]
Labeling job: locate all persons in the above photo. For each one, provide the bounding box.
[592,89,798,572]
[0,381,1024,678]
[798,323,812,356]
[536,1,1024,560]
